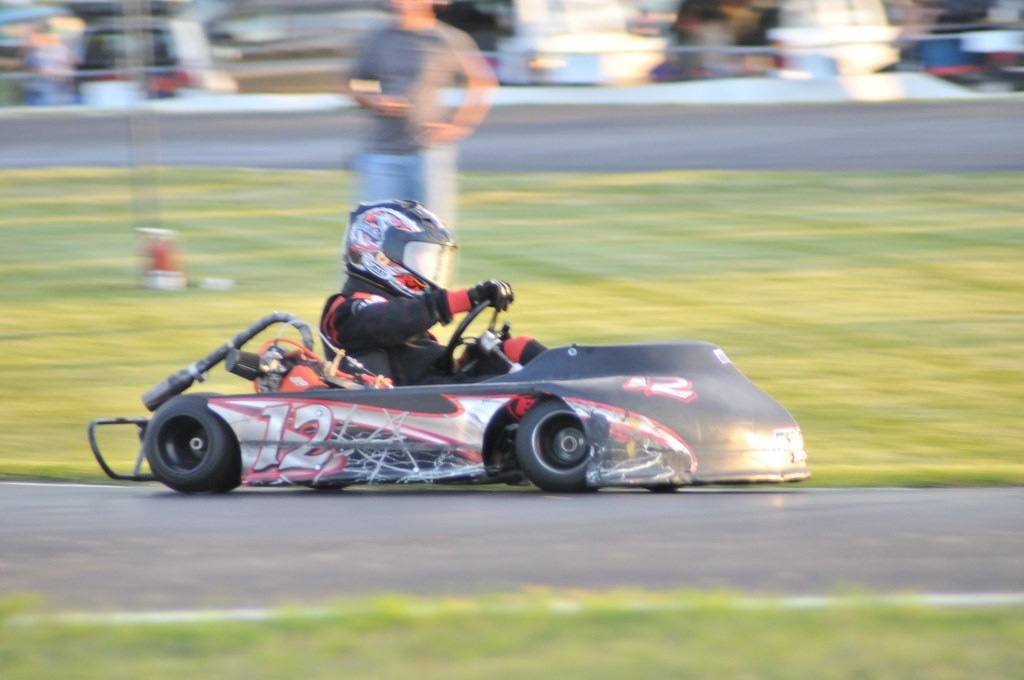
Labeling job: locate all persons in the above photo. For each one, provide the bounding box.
[647,0,784,82]
[870,1,1024,92]
[1,2,238,107]
[318,198,546,386]
[432,1,519,85]
[335,0,498,289]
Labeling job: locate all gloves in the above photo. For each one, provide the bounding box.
[468,280,514,312]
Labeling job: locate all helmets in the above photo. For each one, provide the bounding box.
[343,199,458,299]
